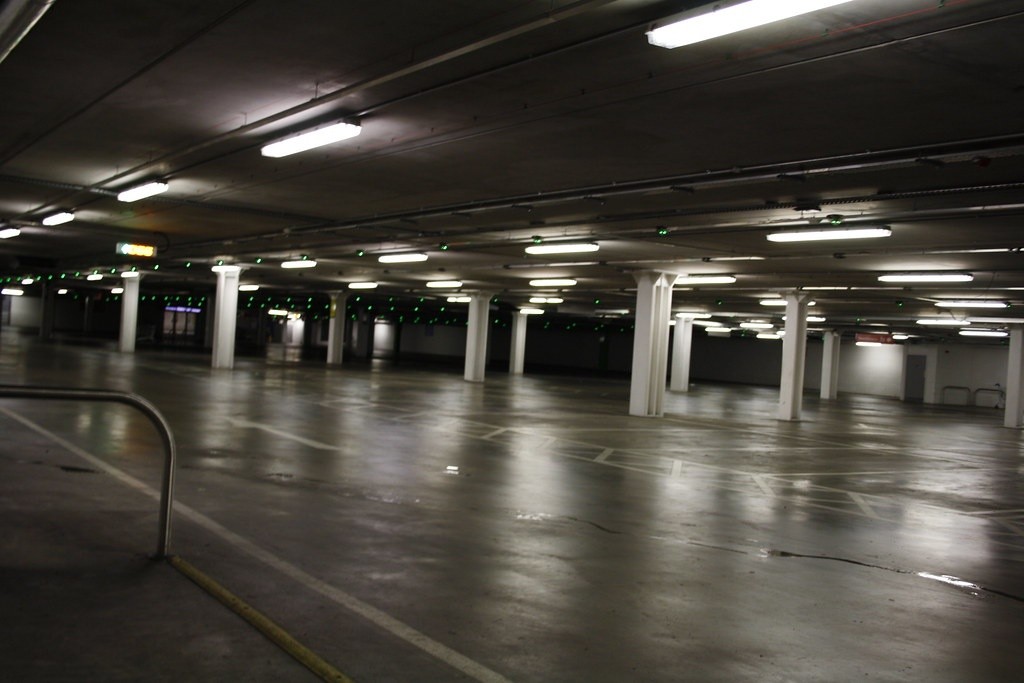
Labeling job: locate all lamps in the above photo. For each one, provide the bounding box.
[767,224,892,243]
[877,272,975,282]
[646,1,852,49]
[259,115,361,158]
